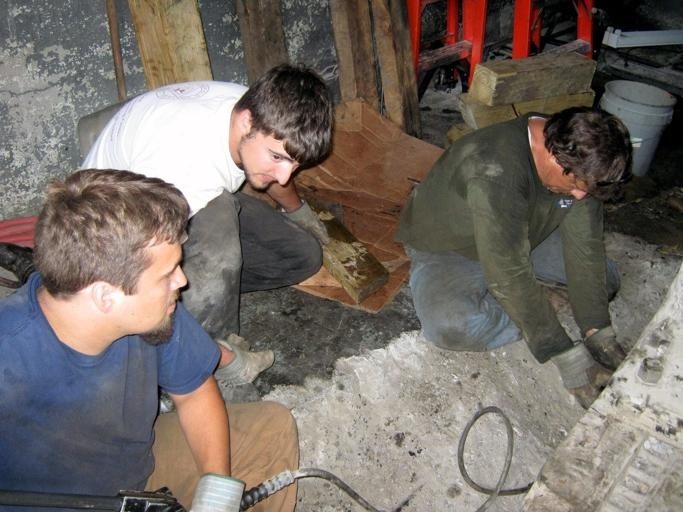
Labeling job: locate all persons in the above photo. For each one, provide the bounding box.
[0,168,299,512]
[394,105,632,412]
[83,64,334,387]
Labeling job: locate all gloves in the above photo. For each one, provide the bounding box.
[279,198,329,246]
[584,325,627,371]
[550,339,615,410]
[214,332,275,385]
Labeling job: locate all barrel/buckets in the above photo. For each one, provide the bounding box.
[600,80,676,178]
